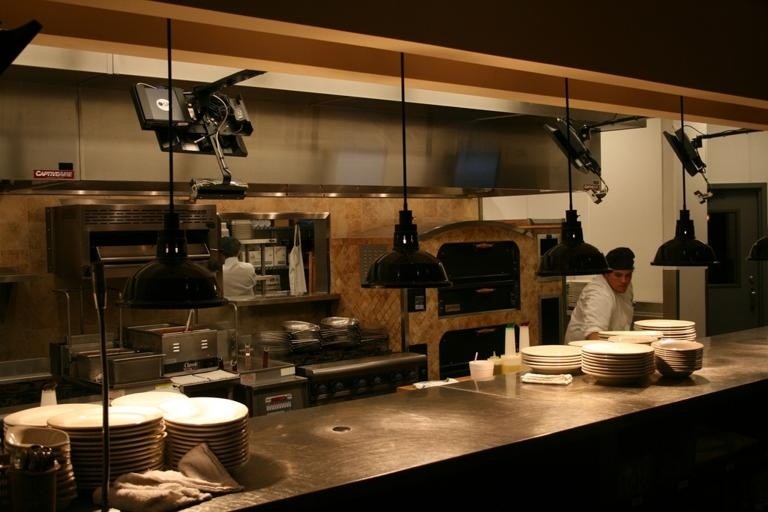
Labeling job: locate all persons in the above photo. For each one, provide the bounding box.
[563,247,635,345]
[221,236,258,297]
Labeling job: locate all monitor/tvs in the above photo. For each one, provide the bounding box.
[130,83,253,157]
[663,128,707,176]
[546,117,600,173]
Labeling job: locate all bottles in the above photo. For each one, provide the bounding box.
[504,318,531,355]
[439,296,445,314]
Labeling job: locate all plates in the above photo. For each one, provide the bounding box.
[230,219,252,241]
[522,318,704,383]
[3,389,248,497]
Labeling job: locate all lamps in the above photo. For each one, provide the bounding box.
[650,95,717,266]
[123,19,228,309]
[534,78,613,276]
[361,52,453,289]
[746,235,768,260]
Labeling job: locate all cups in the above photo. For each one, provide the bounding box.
[468,360,495,381]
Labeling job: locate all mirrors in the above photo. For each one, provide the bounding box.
[707,207,741,289]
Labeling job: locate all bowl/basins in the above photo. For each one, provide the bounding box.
[256,315,388,356]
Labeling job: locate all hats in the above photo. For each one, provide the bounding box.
[606,247,635,270]
[222,238,241,255]
